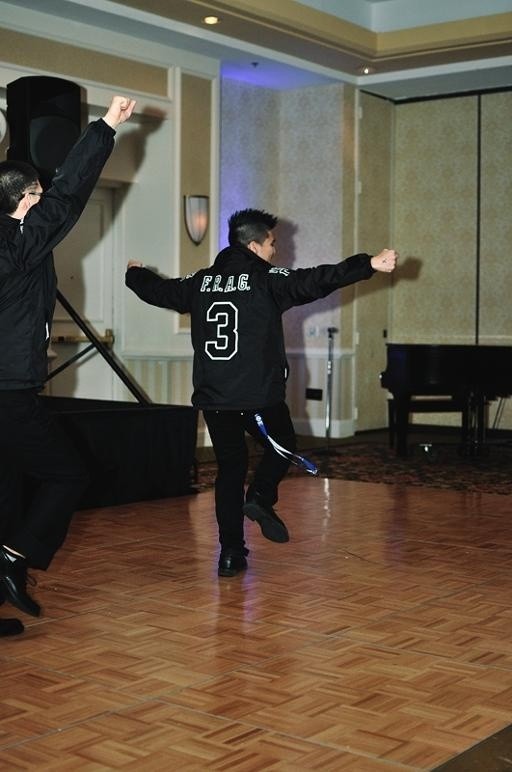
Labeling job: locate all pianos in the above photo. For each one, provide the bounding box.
[379,343,511,457]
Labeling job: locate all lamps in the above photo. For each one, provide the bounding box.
[184,193,210,245]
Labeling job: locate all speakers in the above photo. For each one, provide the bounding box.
[6,76,88,191]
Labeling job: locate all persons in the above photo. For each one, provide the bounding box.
[129,206,397,576]
[0,96,137,633]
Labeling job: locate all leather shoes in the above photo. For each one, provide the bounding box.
[0,618,25,639]
[217,554,248,577]
[242,496,289,543]
[0,549,41,617]
